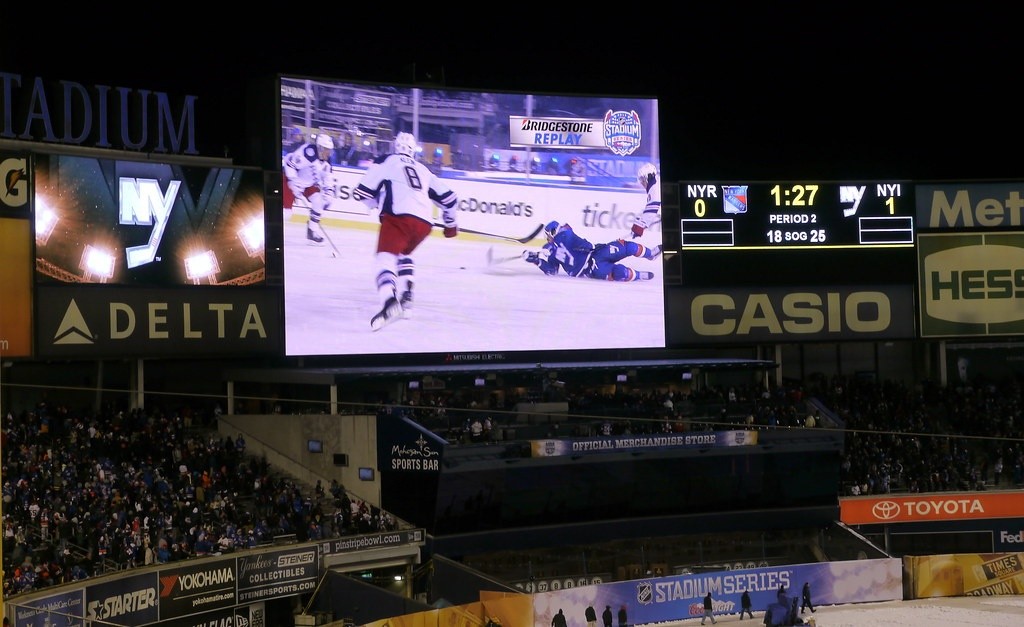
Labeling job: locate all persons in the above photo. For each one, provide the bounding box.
[401,378,816,439]
[1,390,402,600]
[830,387,1024,498]
[602,605,613,627]
[551,608,567,627]
[801,582,817,613]
[740,591,753,620]
[776,585,786,601]
[993,458,1003,484]
[701,592,717,624]
[585,603,597,627]
[617,605,628,627]
[282,124,662,331]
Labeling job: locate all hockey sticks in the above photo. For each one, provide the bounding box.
[433,222,545,245]
[594,220,661,247]
[304,201,342,259]
[487,248,522,266]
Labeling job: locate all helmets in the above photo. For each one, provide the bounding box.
[637,163,657,187]
[317,134,334,150]
[544,220,561,239]
[394,131,415,157]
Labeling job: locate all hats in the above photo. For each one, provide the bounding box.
[606,605,611,608]
[559,609,563,612]
[744,589,748,592]
[589,603,593,607]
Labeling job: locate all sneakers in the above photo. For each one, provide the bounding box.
[649,244,662,260]
[397,286,414,317]
[634,269,654,281]
[366,296,400,331]
[307,222,324,243]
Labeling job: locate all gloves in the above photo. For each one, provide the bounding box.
[288,181,303,193]
[442,226,458,237]
[523,250,539,264]
[631,223,644,239]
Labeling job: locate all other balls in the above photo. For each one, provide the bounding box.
[460,267,465,269]
